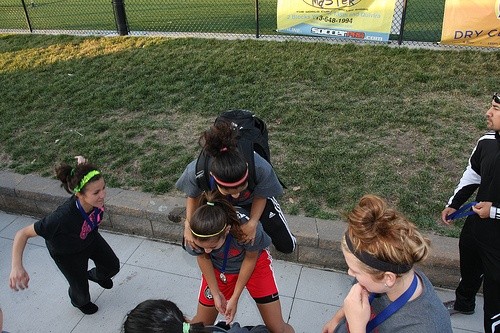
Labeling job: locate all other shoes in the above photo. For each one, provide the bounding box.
[442,300,474,315]
[87,270,113,289]
[71,300,98,315]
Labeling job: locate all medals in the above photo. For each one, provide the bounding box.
[219,273,227,284]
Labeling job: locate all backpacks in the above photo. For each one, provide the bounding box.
[195,110,270,198]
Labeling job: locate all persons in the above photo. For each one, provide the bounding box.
[123,299,271,333]
[8,163,120,315]
[185,190,295,333]
[173,120,297,256]
[323,194,453,333]
[440,92,500,333]
